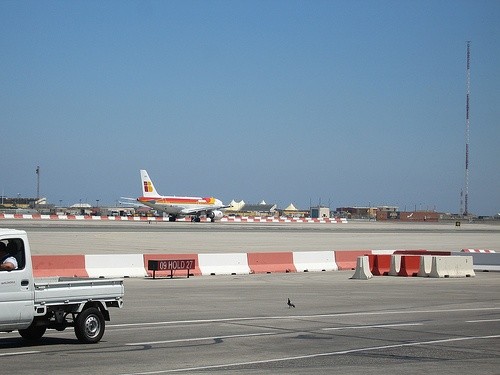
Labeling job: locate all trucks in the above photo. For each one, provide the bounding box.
[0,228,125,344]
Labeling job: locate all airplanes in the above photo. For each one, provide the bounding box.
[136,170,234,222]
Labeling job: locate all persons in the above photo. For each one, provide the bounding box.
[0,242,18,270]
[7,242,22,268]
